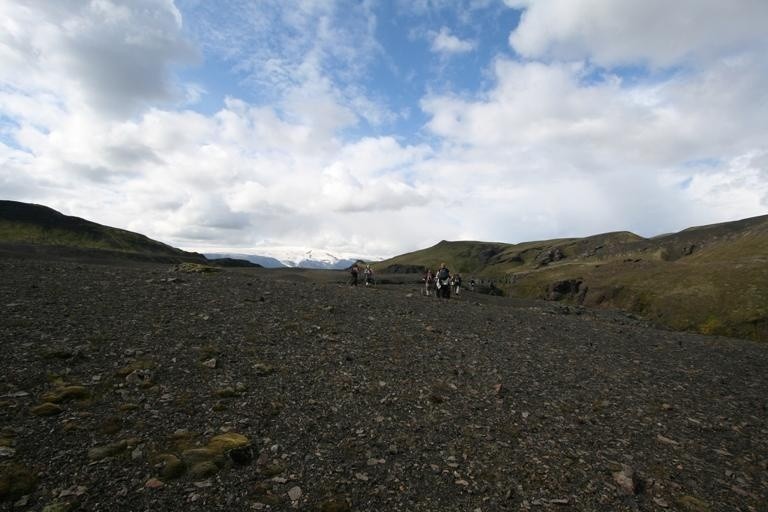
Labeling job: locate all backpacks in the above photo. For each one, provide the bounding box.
[437,268,448,280]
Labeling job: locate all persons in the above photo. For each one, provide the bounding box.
[363,263,372,288]
[349,264,358,286]
[422,262,462,300]
